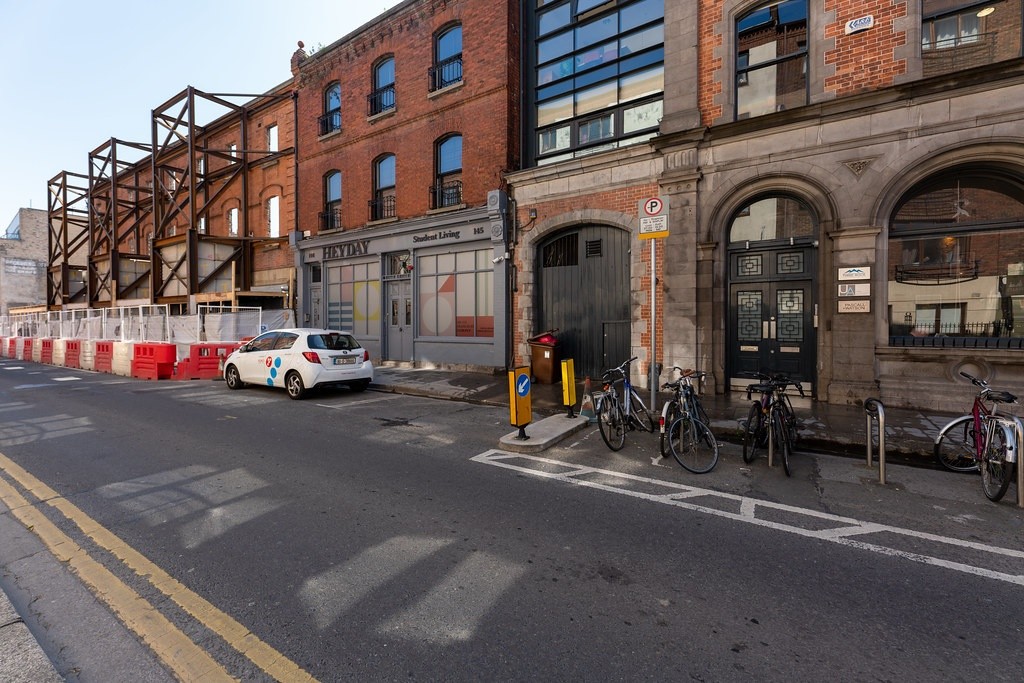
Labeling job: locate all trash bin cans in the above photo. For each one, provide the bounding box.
[527,328,560,385]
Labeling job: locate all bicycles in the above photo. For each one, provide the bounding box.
[742,371,793,464]
[933,371,1019,503]
[746,381,804,478]
[591,356,655,453]
[660,366,707,458]
[661,370,719,475]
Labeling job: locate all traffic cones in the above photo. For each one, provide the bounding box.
[580,376,598,423]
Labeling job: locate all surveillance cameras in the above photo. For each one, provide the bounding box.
[493,257,501,264]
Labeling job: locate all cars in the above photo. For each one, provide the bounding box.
[223,327,374,400]
